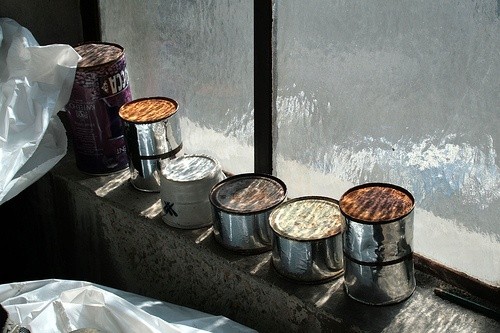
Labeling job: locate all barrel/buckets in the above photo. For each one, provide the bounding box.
[56,38,131,178]
[338,178,416,306]
[209,172,287,250]
[116,97,182,193]
[157,153,222,229]
[268,193,344,283]
